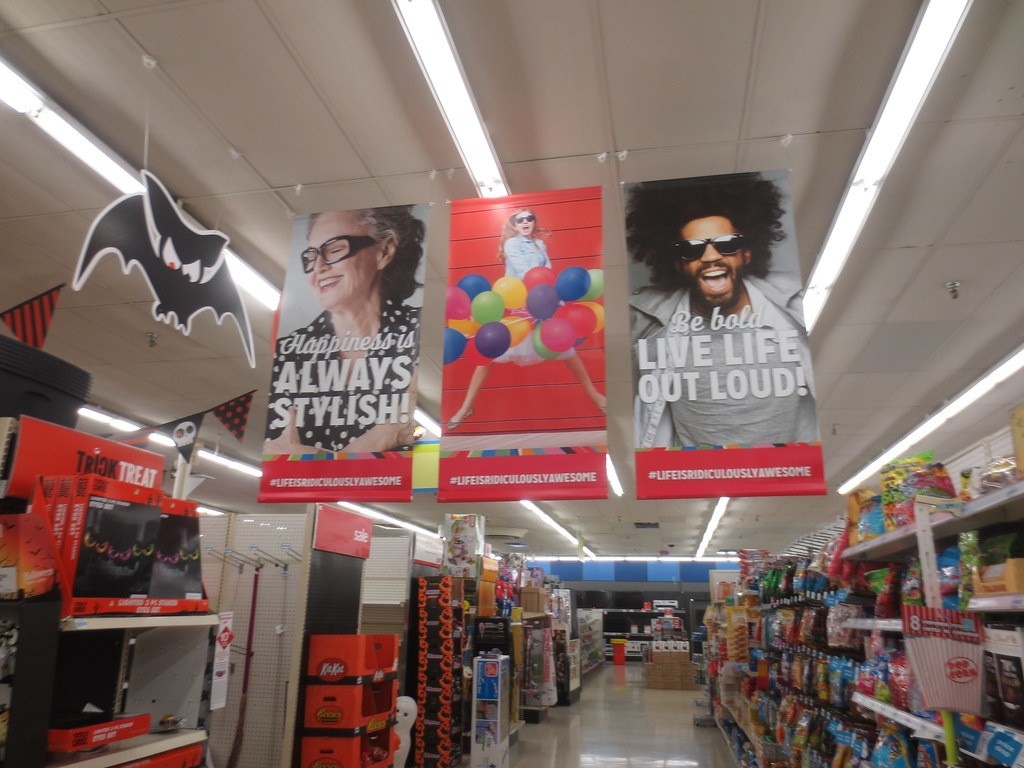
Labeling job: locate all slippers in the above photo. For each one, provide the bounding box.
[598,406,608,415]
[447,407,474,430]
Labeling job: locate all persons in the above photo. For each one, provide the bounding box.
[262,205,427,456]
[622,171,820,448]
[446,207,608,431]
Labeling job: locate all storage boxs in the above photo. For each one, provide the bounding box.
[520,587,546,613]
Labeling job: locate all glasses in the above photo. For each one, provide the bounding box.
[672,233,744,261]
[301,236,375,274]
[515,215,535,224]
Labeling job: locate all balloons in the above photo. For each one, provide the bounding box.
[443,265,605,368]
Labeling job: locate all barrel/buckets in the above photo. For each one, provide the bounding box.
[611,639,627,665]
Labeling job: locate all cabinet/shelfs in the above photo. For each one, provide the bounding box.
[0,407,220,768]
[604,609,688,662]
[837,478,1024,766]
[578,608,607,679]
[708,523,836,766]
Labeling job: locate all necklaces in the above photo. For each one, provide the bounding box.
[331,291,380,336]
